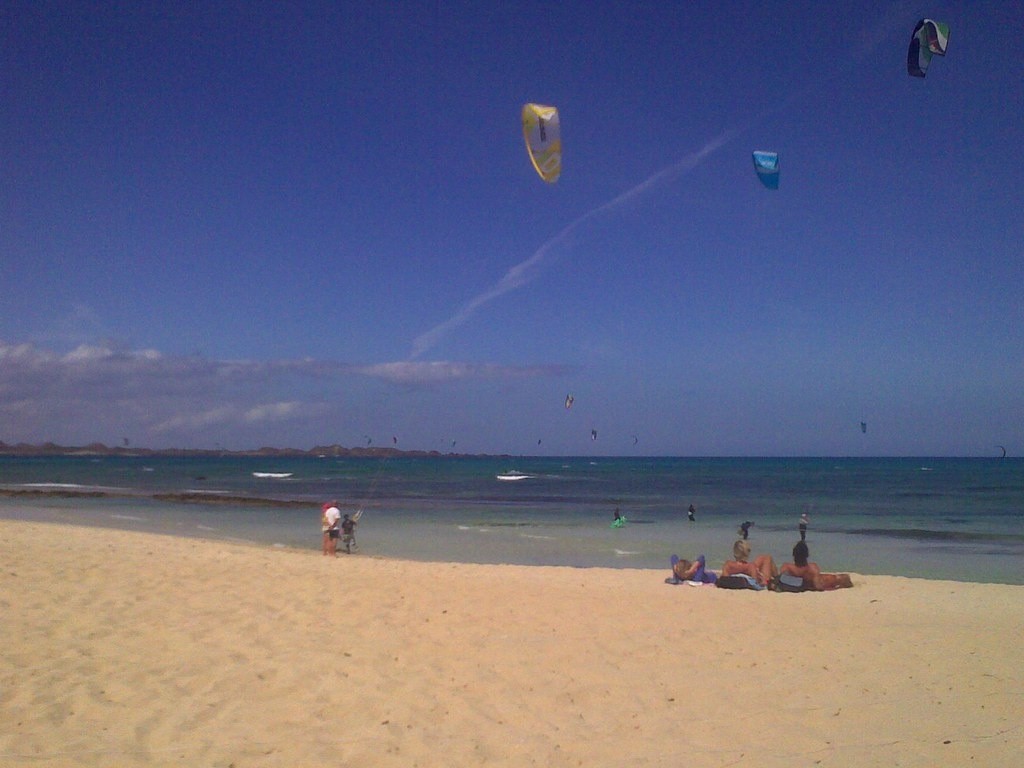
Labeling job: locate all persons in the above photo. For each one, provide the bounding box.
[741,520,755,539]
[799,513,809,541]
[721,540,778,591]
[670,553,717,584]
[688,504,695,521]
[341,514,358,554]
[614,508,621,521]
[321,500,341,556]
[780,541,853,592]
